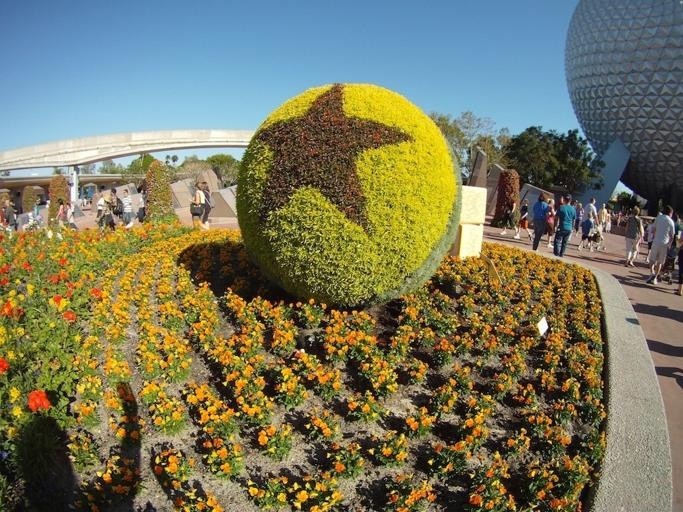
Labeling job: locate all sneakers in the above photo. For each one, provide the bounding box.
[625,259,635,266]
[647,272,683,284]
[546,243,554,248]
[500,230,534,241]
[674,288,681,295]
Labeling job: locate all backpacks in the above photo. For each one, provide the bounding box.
[204,190,215,208]
[113,197,123,214]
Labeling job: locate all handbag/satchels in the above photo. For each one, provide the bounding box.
[190,203,206,215]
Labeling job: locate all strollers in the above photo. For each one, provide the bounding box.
[656,250,674,285]
[587,226,607,252]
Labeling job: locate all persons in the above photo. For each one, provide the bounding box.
[95,189,107,235]
[498,191,683,296]
[121,189,132,231]
[188,182,206,228]
[109,188,120,230]
[33,202,39,216]
[66,203,76,228]
[55,204,65,228]
[137,186,147,223]
[4,199,18,226]
[202,182,212,230]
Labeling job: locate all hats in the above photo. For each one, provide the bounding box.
[103,193,112,203]
